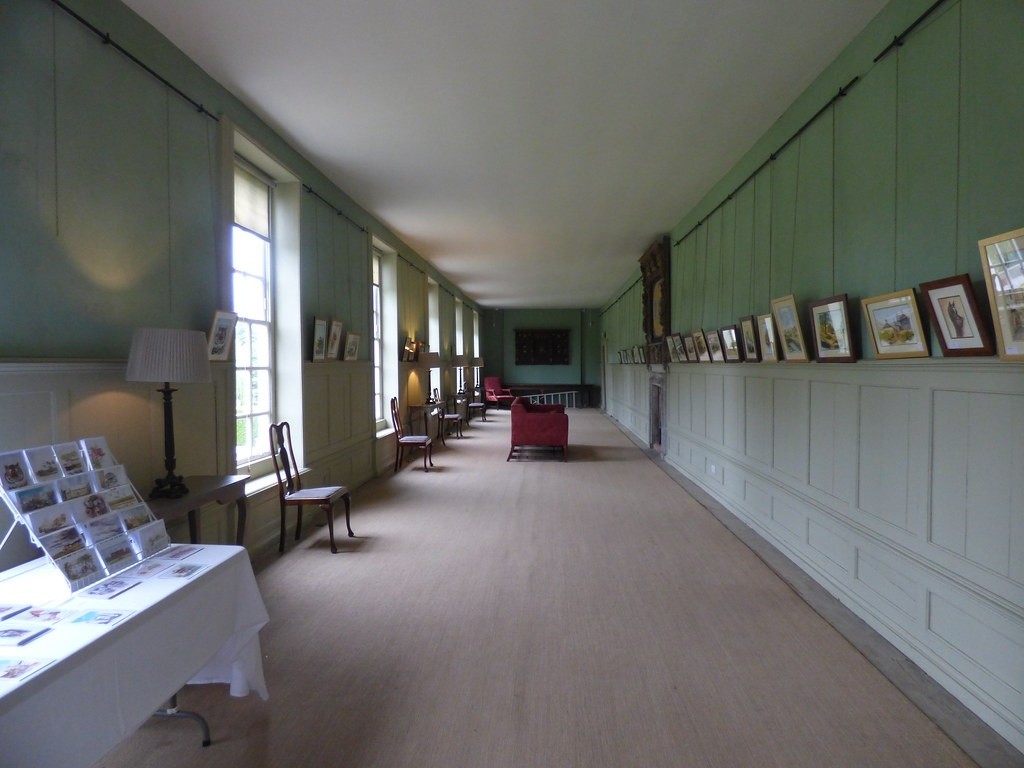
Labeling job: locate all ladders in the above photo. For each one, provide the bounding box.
[519,390,581,411]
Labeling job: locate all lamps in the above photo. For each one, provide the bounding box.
[452,355,469,394]
[125,326,213,500]
[471,357,485,388]
[418,352,442,404]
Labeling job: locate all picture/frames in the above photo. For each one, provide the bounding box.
[312,315,329,362]
[738,315,760,363]
[343,332,361,362]
[327,321,342,359]
[860,287,931,359]
[683,336,699,363]
[704,329,726,363]
[401,337,430,363]
[617,345,646,364]
[690,328,712,363]
[770,293,810,364]
[758,313,779,364]
[671,332,689,363]
[808,293,857,364]
[720,324,742,363]
[918,273,995,358]
[207,309,238,362]
[666,336,680,363]
[977,228,1024,362]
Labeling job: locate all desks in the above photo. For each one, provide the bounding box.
[407,401,448,454]
[470,388,486,414]
[446,395,470,429]
[142,473,251,546]
[0,542,270,768]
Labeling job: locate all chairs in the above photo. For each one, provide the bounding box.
[268,421,355,555]
[465,387,487,422]
[389,396,434,473]
[432,386,464,441]
[484,377,514,410]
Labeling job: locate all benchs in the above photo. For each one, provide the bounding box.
[506,396,569,462]
